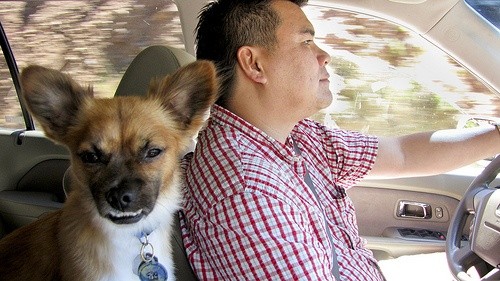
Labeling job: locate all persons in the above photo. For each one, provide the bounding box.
[179,0,500,281]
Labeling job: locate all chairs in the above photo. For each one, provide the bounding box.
[114,44,196,281]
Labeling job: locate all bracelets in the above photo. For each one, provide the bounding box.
[492,122,500,134]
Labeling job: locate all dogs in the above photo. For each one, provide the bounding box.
[0,60,220,281]
